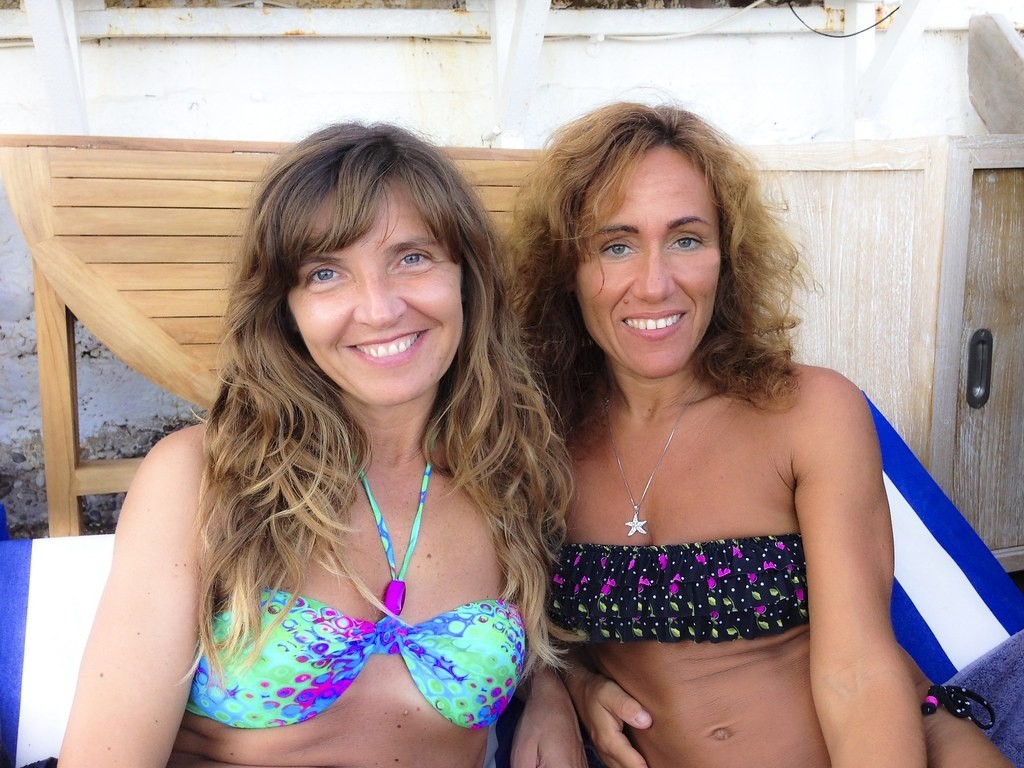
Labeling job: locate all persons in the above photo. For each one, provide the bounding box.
[510,105,1018,768]
[55,124,590,768]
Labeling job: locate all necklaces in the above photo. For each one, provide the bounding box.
[602,387,699,538]
